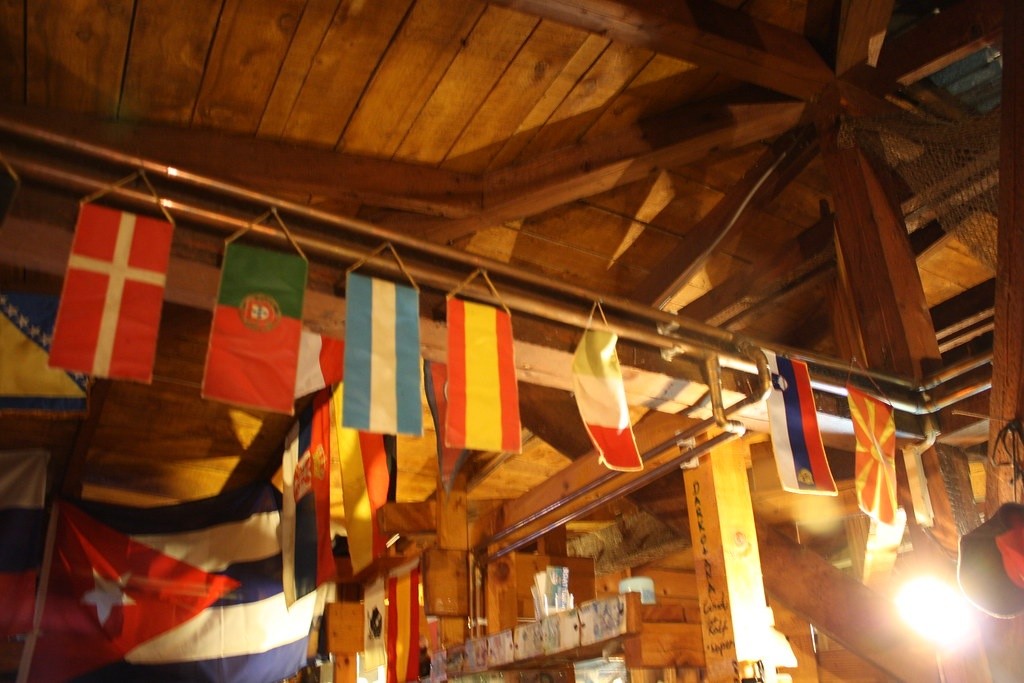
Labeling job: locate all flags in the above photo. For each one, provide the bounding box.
[0,291,93,417]
[846,385,898,526]
[282,331,465,683]
[760,349,839,496]
[200,242,309,416]
[47,202,174,385]
[0,448,50,636]
[445,295,522,455]
[572,329,643,471]
[342,273,423,436]
[22,477,316,683]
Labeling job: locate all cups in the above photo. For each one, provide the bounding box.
[619,576,655,605]
